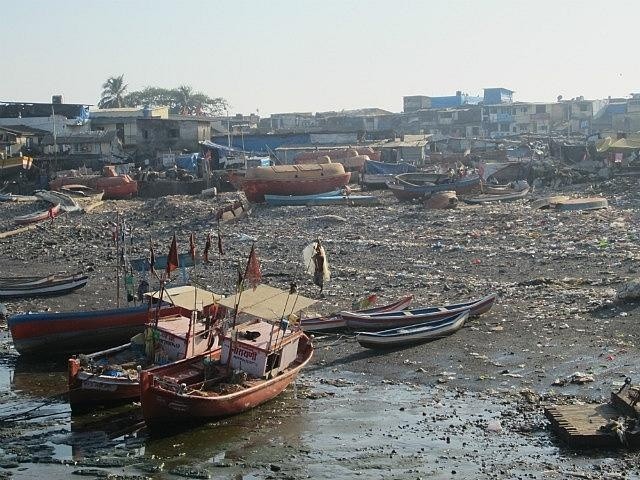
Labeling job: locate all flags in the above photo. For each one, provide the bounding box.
[112,221,263,303]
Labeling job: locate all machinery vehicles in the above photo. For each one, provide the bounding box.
[0,129,34,196]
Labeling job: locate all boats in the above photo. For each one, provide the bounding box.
[8,292,171,357]
[353,310,474,348]
[135,243,316,432]
[68,236,230,420]
[12,202,64,225]
[1,272,89,298]
[301,296,415,331]
[369,155,552,201]
[342,296,497,334]
